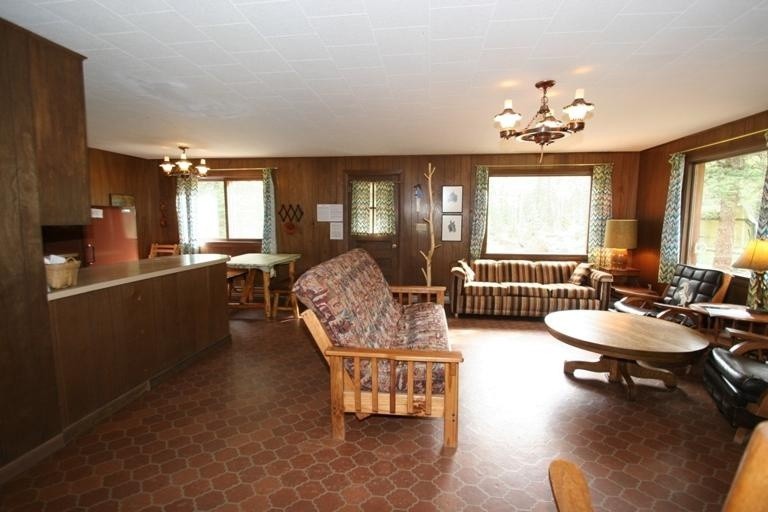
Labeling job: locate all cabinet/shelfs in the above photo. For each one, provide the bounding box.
[26,24,94,228]
[0,16,66,491]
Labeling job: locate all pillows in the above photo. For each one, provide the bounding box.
[566,259,594,288]
[449,259,474,284]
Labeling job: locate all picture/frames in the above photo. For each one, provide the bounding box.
[441,184,464,214]
[441,214,463,242]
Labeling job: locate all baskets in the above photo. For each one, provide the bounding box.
[45,258,82,289]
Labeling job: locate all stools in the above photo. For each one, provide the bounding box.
[226,269,250,304]
[269,273,300,319]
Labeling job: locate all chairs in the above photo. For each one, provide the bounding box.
[699,336,767,446]
[608,261,735,339]
[147,242,184,259]
[541,421,767,512]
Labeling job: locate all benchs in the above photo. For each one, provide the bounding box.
[286,244,465,452]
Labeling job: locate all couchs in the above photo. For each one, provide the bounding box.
[447,253,609,327]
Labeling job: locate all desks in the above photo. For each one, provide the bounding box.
[227,248,303,319]
[691,298,763,347]
[611,280,660,313]
[598,265,641,308]
[537,306,709,402]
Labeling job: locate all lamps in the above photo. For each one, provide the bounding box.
[729,237,767,313]
[156,143,212,182]
[601,218,639,269]
[488,78,597,164]
